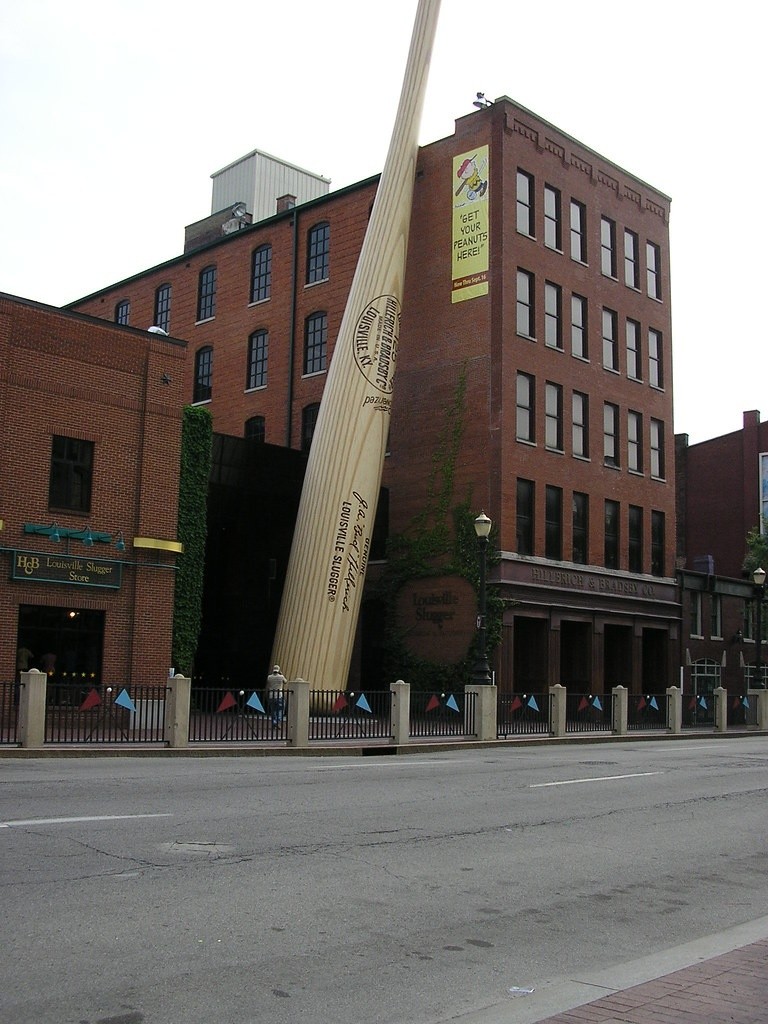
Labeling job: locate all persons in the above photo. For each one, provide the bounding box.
[265,665,287,730]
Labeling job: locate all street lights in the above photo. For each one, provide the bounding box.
[471,510,493,685]
[753,567,767,688]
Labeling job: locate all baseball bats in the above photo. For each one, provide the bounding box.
[263,0,443,712]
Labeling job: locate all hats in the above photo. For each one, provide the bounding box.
[273,665,280,672]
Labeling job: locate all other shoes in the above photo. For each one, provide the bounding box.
[272,721,281,730]
